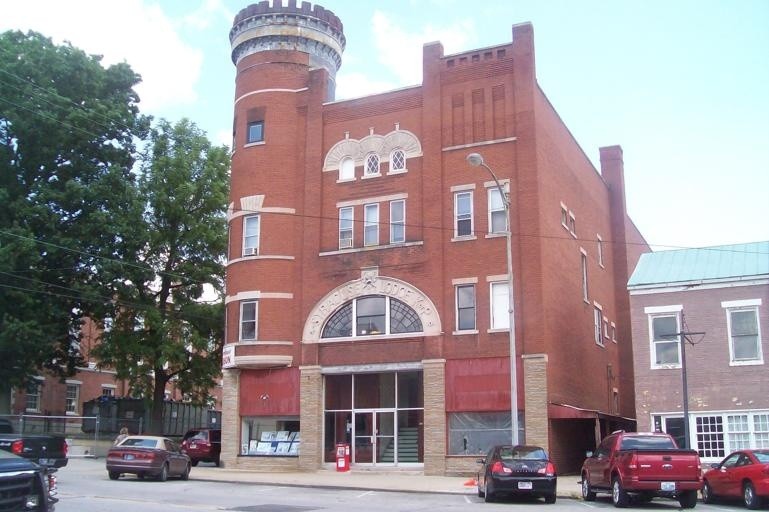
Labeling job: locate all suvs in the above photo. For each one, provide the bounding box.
[0,447,58,511]
[181,426,221,466]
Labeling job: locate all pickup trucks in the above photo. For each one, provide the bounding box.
[577,429,701,511]
[0,417,68,474]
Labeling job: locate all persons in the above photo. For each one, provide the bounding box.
[113,426,130,477]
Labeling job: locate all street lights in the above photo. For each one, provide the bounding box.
[466,152,519,446]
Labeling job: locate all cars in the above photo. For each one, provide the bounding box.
[104,435,192,481]
[475,443,560,504]
[701,447,769,510]
